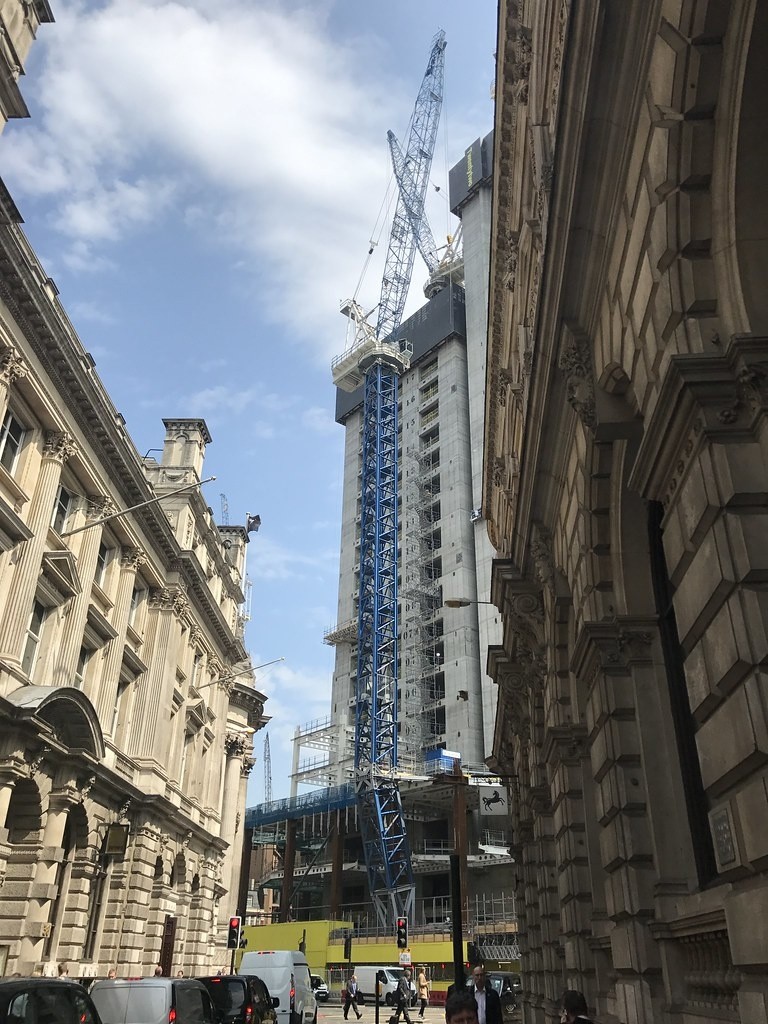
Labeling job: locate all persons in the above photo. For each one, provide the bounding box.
[31,971,41,977]
[108,969,116,980]
[418,967,430,1020]
[559,990,594,1024]
[178,971,183,977]
[465,966,504,1024]
[344,975,362,1020]
[153,967,162,977]
[219,968,227,975]
[395,970,414,1024]
[445,991,479,1024]
[58,963,68,977]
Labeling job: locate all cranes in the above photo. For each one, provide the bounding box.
[387,129,464,298]
[262,731,274,845]
[337,21,446,931]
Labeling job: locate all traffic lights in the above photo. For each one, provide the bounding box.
[227,916,240,950]
[397,917,408,948]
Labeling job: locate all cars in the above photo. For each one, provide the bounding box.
[0,979,104,1024]
[195,974,281,1024]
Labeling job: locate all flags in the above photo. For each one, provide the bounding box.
[247,515,261,533]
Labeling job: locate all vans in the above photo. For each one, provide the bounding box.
[465,972,523,1001]
[239,950,319,1024]
[311,974,331,1002]
[91,976,218,1024]
[353,966,417,1008]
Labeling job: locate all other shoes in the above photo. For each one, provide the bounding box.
[407,1022,413,1024]
[346,1018,348,1020]
[357,1014,362,1019]
